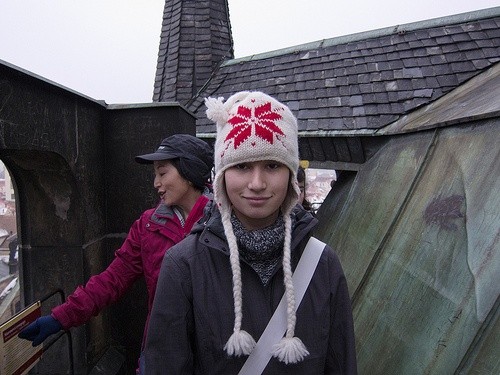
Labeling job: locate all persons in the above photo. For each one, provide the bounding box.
[139,91,357,375]
[297,166,316,218]
[18,134,214,347]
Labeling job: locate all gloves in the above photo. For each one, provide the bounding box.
[18,315,64,347]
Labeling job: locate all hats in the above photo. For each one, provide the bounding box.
[134,134,214,178]
[204,91,301,216]
